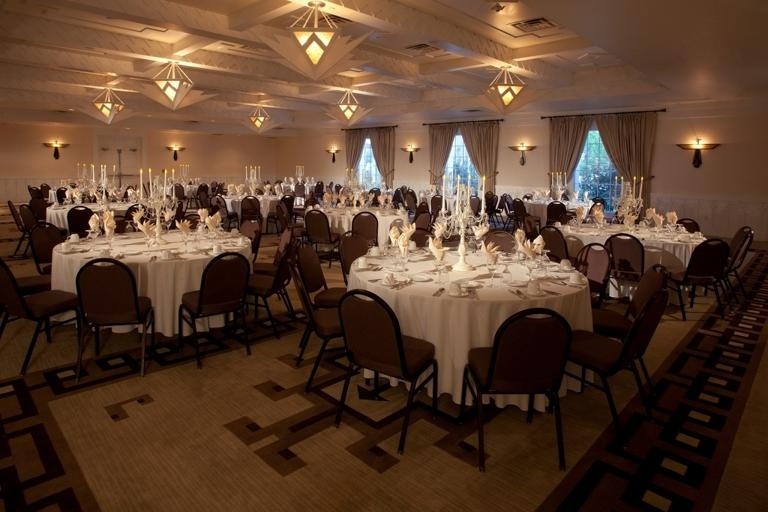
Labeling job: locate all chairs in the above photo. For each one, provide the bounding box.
[1,257,80,375]
[178,250,251,370]
[0,183,754,344]
[76,258,155,376]
[549,289,671,443]
[458,308,573,470]
[289,260,342,391]
[334,289,439,454]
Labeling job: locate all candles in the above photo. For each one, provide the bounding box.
[550,170,569,190]
[613,175,644,205]
[244,164,262,183]
[439,172,487,215]
[139,162,192,201]
[75,162,116,183]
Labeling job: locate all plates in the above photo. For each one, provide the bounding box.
[58,228,248,262]
[311,205,402,216]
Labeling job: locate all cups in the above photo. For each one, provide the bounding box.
[550,221,704,239]
[358,243,584,296]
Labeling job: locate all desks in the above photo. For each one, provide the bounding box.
[347,249,593,413]
[53,229,251,357]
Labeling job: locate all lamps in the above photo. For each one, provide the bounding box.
[472,65,547,116]
[166,143,186,161]
[133,59,218,113]
[325,145,342,163]
[508,141,538,166]
[259,1,374,81]
[74,88,143,127]
[240,104,281,135]
[322,90,375,129]
[400,143,421,164]
[675,137,721,169]
[42,140,71,159]
